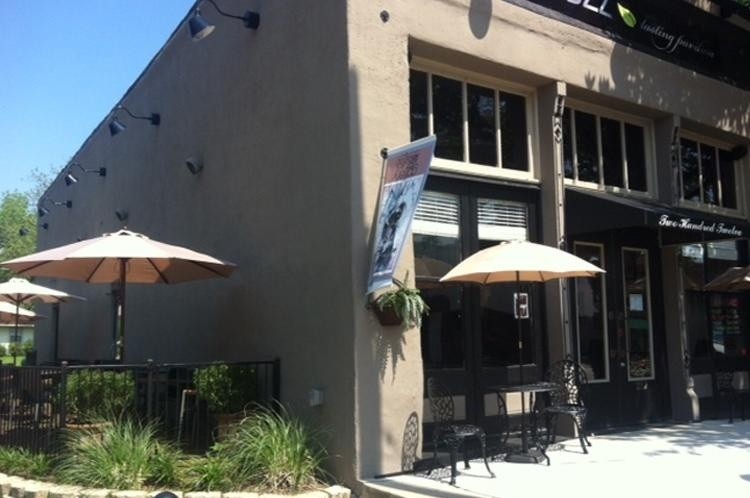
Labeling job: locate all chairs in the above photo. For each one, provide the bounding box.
[543,359,592,455]
[427,377,496,485]
[710,352,748,423]
[8,367,54,454]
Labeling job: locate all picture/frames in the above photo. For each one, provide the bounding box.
[514,293,530,320]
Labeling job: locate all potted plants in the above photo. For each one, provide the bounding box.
[64,368,136,448]
[193,360,251,443]
[365,270,431,329]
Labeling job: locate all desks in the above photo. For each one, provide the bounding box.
[485,382,561,465]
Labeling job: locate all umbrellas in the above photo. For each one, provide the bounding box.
[0,225,240,371]
[0,301,48,325]
[700,264,750,296]
[438,240,609,453]
[0,278,87,365]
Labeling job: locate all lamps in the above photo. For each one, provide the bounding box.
[64,163,106,187]
[20,219,49,236]
[189,0,259,42]
[109,107,161,137]
[37,196,73,217]
